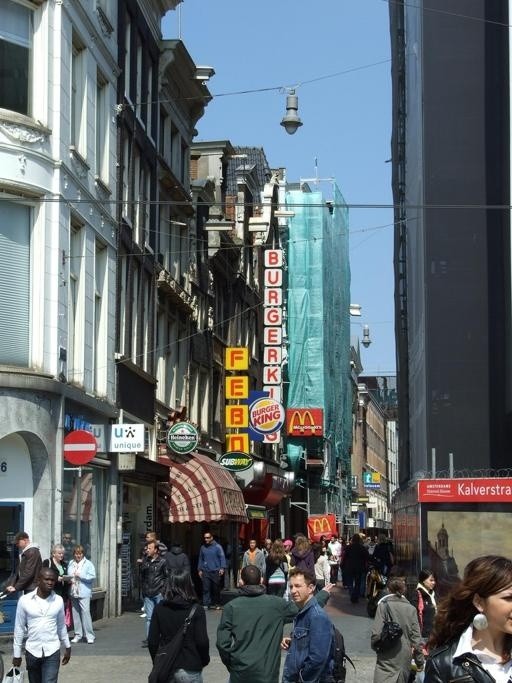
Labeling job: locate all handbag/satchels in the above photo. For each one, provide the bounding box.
[371,600,403,654]
[3,666,24,683]
[147,615,191,682]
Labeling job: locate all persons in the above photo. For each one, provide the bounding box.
[0,530,512,683]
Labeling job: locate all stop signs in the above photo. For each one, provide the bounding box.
[62,429,97,464]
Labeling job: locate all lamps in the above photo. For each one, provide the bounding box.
[360,326,372,348]
[113,88,304,135]
[160,214,188,226]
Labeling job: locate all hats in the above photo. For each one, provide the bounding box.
[10,531,28,545]
[282,539,292,547]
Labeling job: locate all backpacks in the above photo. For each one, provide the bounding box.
[332,628,347,683]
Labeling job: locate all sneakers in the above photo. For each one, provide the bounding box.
[70,637,95,644]
[139,605,147,618]
[203,605,221,611]
[141,639,150,649]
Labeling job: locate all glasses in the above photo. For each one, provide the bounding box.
[204,537,211,539]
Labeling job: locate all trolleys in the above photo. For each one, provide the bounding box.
[0,587,12,682]
